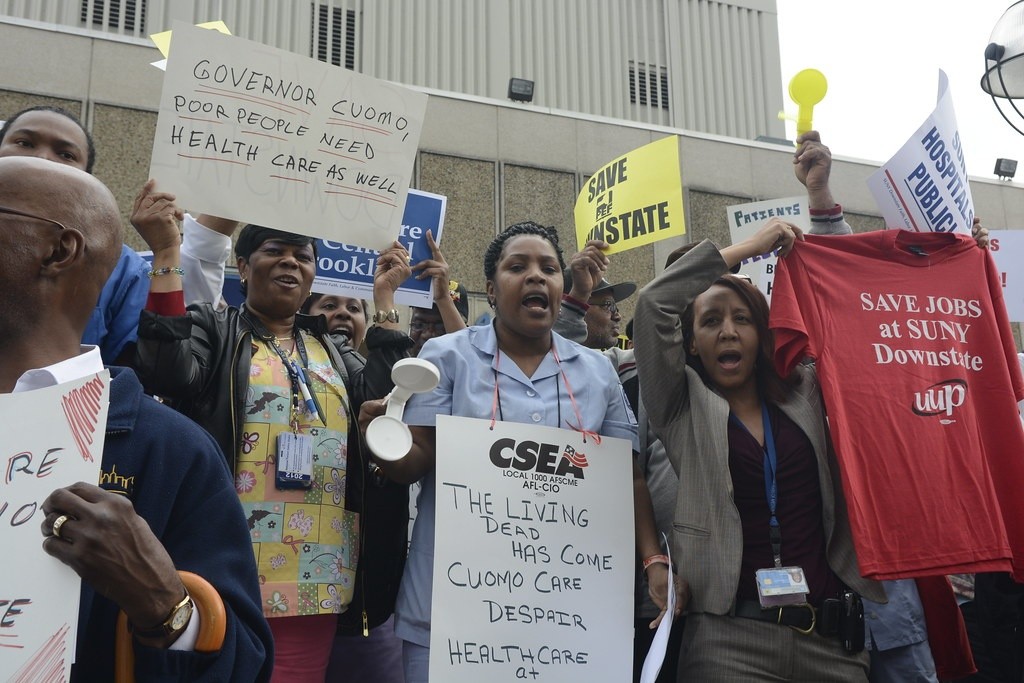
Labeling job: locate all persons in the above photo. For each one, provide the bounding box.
[787,569,802,583]
[0,104,1024,682]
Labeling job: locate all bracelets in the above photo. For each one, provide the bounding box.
[642,554,675,579]
[147,267,184,278]
[372,309,400,323]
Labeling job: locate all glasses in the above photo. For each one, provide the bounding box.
[407,321,446,337]
[587,300,619,313]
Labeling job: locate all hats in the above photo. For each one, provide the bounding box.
[409,280,469,322]
[563,268,636,303]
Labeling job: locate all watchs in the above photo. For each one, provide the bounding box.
[127,582,193,637]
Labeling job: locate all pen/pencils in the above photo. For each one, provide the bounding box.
[302,368,327,426]
[291,361,321,423]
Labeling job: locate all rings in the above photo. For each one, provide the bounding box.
[52,515,77,539]
[406,255,410,262]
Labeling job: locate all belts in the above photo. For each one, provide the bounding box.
[734,599,841,636]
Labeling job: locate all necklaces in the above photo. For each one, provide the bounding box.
[277,336,295,341]
